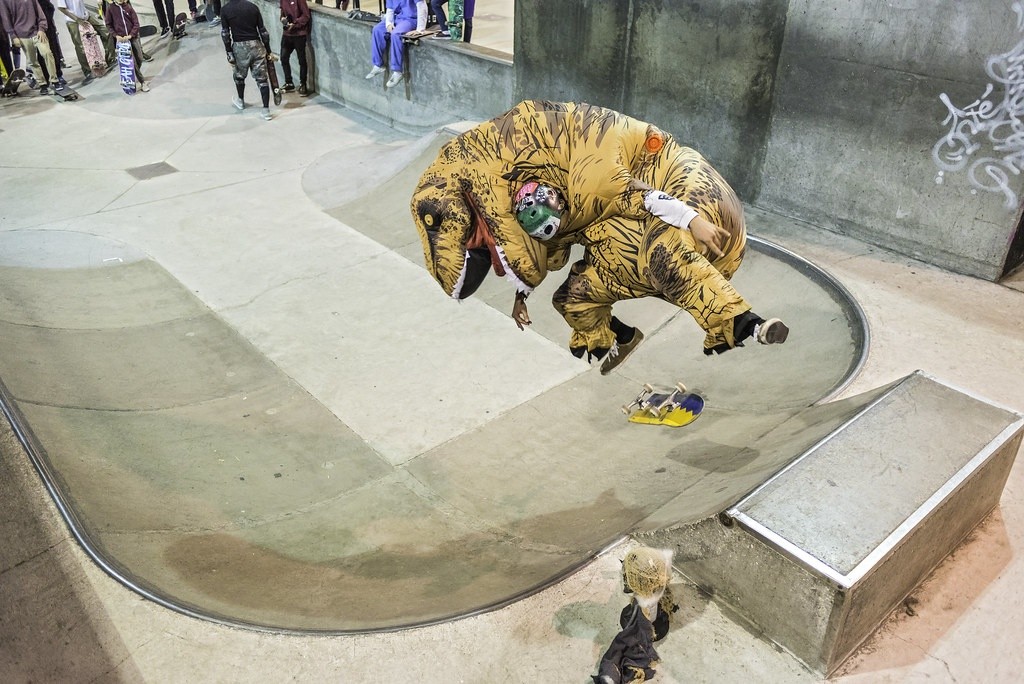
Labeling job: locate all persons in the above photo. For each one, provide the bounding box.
[219,0,274,121]
[0,0,476,101]
[510,179,790,376]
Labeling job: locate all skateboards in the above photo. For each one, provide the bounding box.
[78,20,108,78]
[0,69,25,96]
[399,24,442,45]
[265,52,285,106]
[195,3,205,20]
[139,25,157,38]
[48,82,78,101]
[172,12,187,39]
[621,382,705,428]
[115,39,137,96]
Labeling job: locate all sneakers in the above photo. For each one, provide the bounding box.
[364,65,387,80]
[297,84,308,96]
[599,329,646,375]
[432,32,452,39]
[0,12,224,96]
[260,109,273,120]
[280,83,296,92]
[754,318,791,346]
[232,96,245,108]
[386,74,404,88]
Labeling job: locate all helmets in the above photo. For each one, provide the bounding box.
[515,183,566,238]
